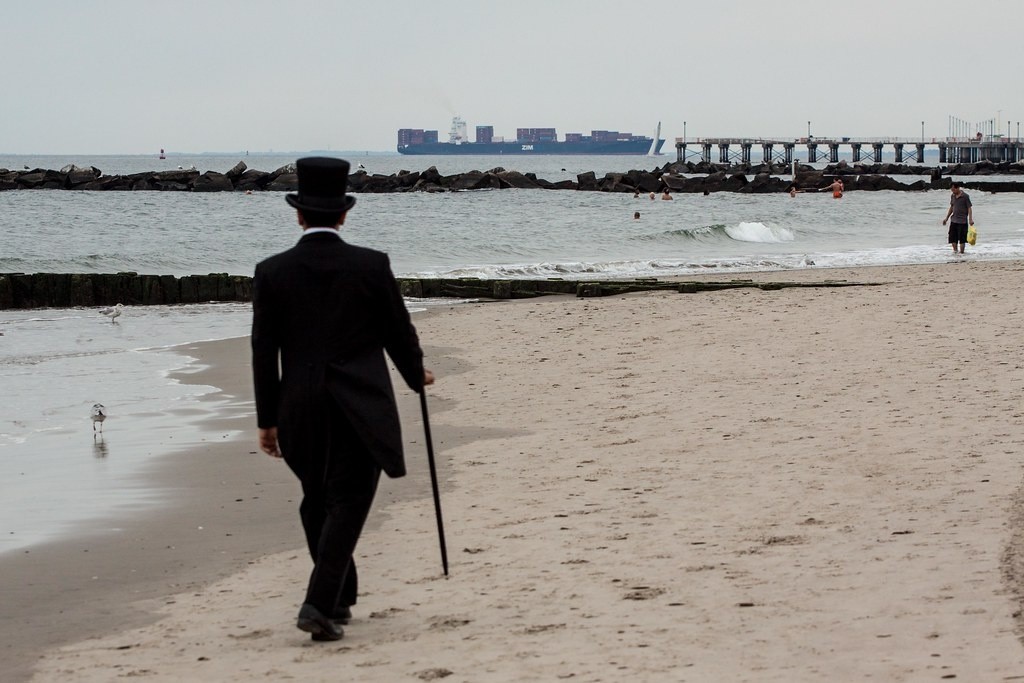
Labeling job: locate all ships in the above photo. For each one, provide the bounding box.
[395,116,666,155]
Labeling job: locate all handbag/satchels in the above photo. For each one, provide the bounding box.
[967,225,976,245]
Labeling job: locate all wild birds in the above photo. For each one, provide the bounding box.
[88,402,107,433]
[98,303,124,324]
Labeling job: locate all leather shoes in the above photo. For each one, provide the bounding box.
[297,605,343,642]
[332,606,351,624]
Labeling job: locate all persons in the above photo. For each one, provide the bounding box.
[634,212,640,219]
[662,189,673,200]
[251,156,434,642]
[790,187,795,197]
[822,178,844,198]
[634,189,639,198]
[943,182,974,253]
[650,192,655,200]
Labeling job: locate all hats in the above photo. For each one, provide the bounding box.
[286,157,355,217]
